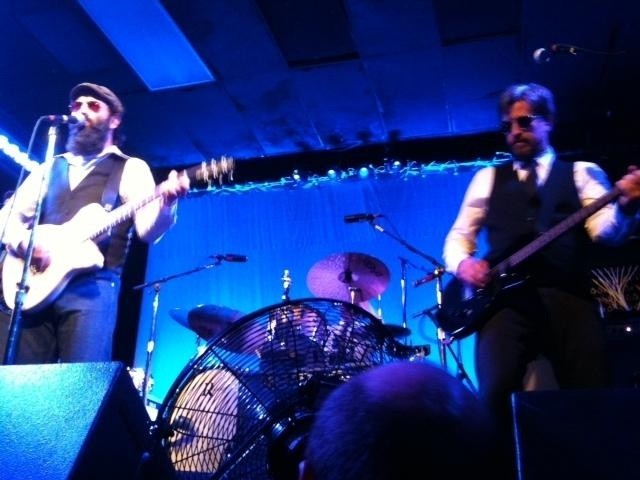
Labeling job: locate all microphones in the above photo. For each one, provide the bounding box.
[532,40,576,62]
[45,112,86,130]
[343,212,375,226]
[209,252,250,264]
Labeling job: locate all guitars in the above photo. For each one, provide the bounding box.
[439,185,619,339]
[2,157,237,313]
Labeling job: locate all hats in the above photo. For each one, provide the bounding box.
[68,81,124,114]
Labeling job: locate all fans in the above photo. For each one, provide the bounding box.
[155,298,395,472]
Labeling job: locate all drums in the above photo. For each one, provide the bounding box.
[169,368,271,475]
[325,320,369,361]
[260,304,327,396]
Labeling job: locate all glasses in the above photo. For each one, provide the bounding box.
[67,100,104,113]
[501,114,546,133]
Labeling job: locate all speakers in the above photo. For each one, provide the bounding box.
[0,360,178,480]
[510,388,640,480]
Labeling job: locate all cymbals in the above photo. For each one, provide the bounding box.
[188,303,270,353]
[168,308,191,328]
[307,253,389,304]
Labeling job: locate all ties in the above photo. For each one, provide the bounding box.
[519,157,540,188]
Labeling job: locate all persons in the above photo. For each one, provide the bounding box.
[0,81,190,365]
[442,83,640,404]
[304,360,499,479]
